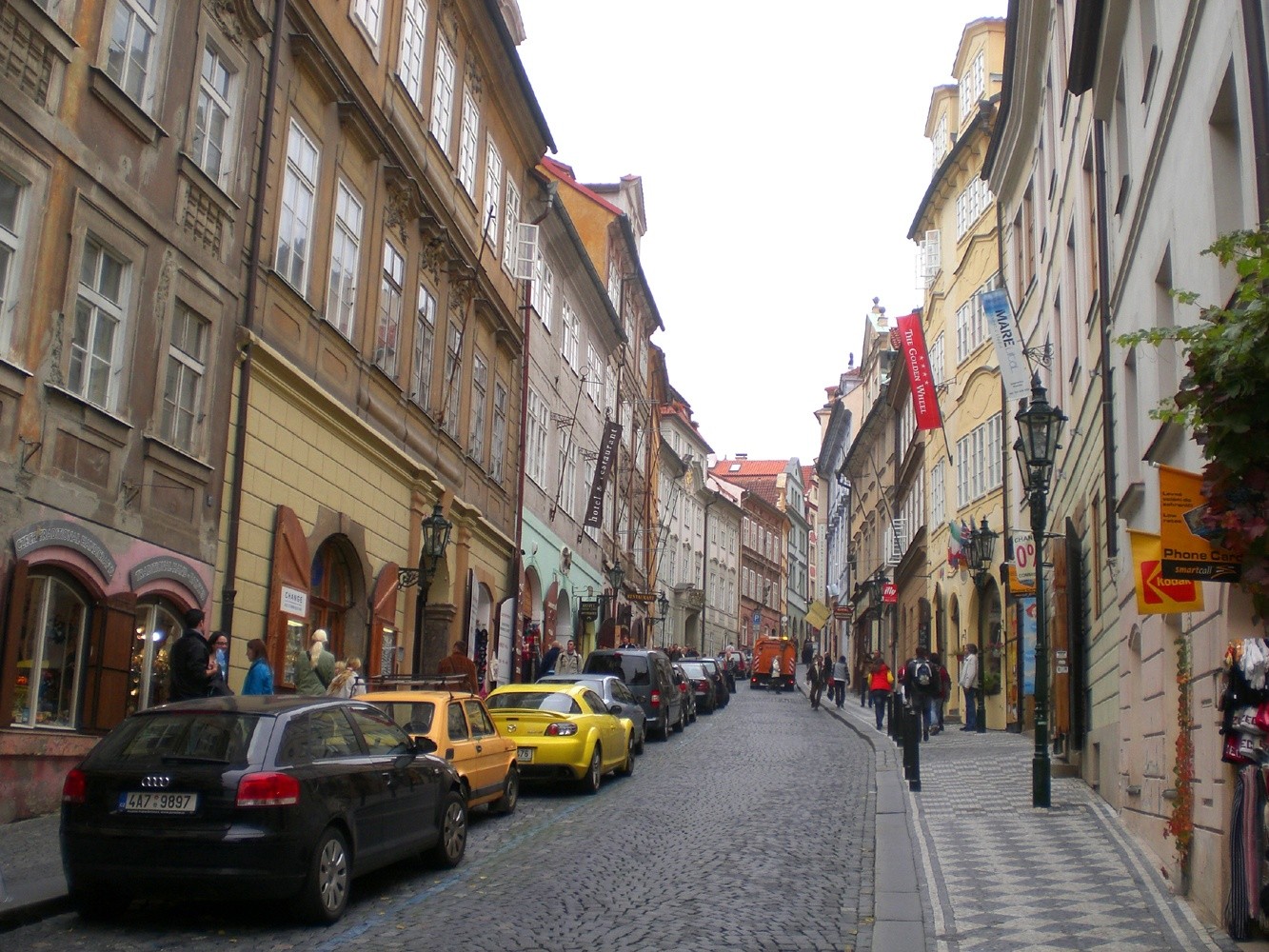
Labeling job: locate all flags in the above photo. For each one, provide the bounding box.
[946,515,979,573]
[980,285,1034,402]
[896,312,943,431]
[583,421,623,529]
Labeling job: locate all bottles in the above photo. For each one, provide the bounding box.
[216,647,225,667]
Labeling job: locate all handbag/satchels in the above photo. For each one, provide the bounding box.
[827,684,835,701]
[769,666,773,672]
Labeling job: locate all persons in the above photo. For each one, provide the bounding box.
[434,641,479,696]
[806,655,834,711]
[725,642,735,651]
[859,650,881,707]
[665,644,699,662]
[810,651,834,691]
[325,657,367,699]
[897,653,951,736]
[169,608,218,702]
[958,643,979,732]
[206,631,235,698]
[833,656,850,709]
[293,629,335,696]
[905,646,939,742]
[766,655,783,694]
[723,651,737,693]
[618,635,635,649]
[868,658,895,730]
[539,639,584,677]
[241,639,274,695]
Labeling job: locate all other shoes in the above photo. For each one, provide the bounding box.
[960,726,976,731]
[939,725,944,731]
[811,700,820,711]
[929,724,940,736]
[869,702,872,708]
[861,704,865,707]
[876,724,883,729]
[838,700,844,708]
[776,691,782,694]
[766,687,769,692]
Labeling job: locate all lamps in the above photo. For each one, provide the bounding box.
[396,499,453,607]
[646,592,669,626]
[597,558,625,600]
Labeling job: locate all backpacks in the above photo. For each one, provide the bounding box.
[932,664,951,702]
[913,659,934,692]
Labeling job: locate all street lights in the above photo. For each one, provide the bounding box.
[1013,370,1068,806]
[962,516,999,733]
[869,569,892,663]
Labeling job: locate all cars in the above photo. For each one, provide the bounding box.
[311,673,520,815]
[525,673,648,757]
[60,692,470,929]
[671,656,736,727]
[468,683,637,796]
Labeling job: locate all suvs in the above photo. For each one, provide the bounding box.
[583,647,686,742]
[716,650,753,680]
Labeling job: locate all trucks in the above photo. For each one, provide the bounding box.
[750,635,798,692]
